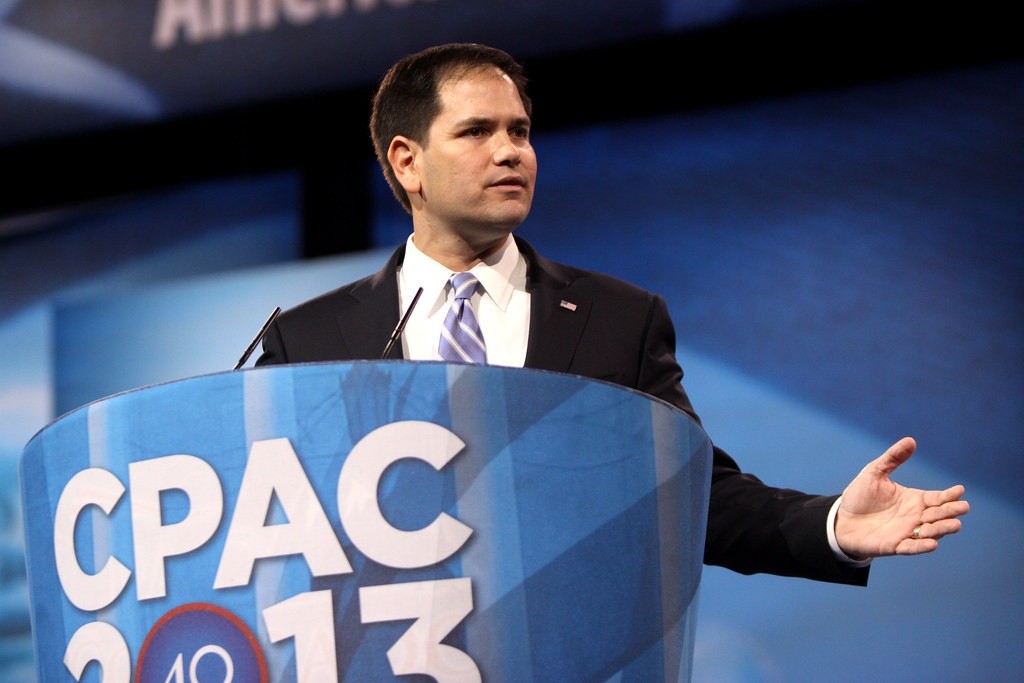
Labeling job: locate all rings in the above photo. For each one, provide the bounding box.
[911,524,920,537]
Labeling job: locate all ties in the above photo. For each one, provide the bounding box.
[438,272,488,365]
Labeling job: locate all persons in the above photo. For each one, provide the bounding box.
[252,43,970,588]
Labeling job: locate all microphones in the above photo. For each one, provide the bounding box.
[381,287,423,359]
[233,307,281,369]
